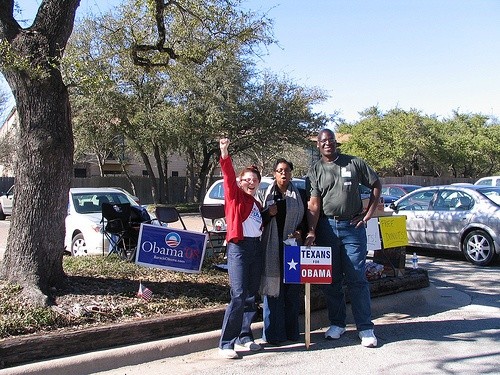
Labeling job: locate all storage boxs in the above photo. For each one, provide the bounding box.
[362,197,406,276]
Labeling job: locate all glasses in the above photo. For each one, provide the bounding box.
[241,177,259,183]
[275,167,291,173]
[320,138,336,143]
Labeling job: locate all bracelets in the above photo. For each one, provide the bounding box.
[295,231,301,235]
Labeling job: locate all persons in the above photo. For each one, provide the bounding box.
[218,139,308,360]
[305,129,382,348]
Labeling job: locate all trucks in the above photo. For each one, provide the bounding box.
[474,176,500,186]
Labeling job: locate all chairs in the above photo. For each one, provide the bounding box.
[200,203,227,263]
[100,202,188,259]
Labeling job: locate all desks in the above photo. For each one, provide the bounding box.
[366,215,407,278]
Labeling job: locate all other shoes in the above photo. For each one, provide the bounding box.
[234,340,261,351]
[218,347,239,359]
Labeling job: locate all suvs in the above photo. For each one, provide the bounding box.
[382,184,432,210]
[203,177,306,231]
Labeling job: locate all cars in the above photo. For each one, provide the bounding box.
[343,173,398,209]
[383,183,500,266]
[0,185,15,220]
[64,187,169,257]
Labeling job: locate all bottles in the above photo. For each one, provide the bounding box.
[413,253,418,269]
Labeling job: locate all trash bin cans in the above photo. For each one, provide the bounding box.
[373,215,407,267]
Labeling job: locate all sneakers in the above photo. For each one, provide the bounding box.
[359,329,377,347]
[324,325,346,339]
